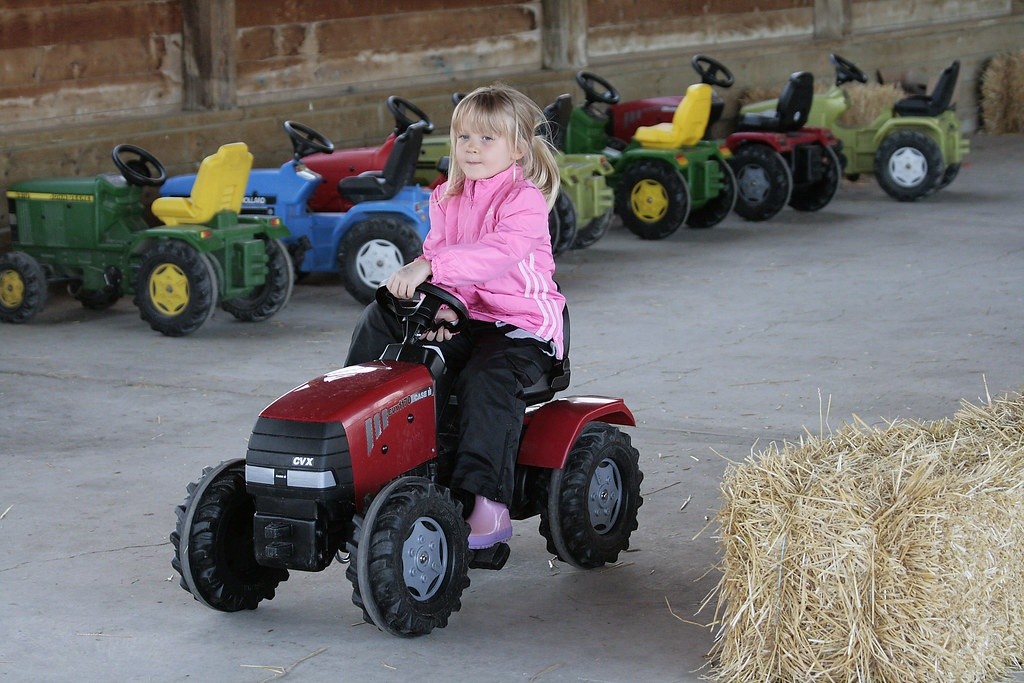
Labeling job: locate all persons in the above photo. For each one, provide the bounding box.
[342,82,567,551]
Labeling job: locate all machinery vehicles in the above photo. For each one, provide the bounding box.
[1,47,968,332]
[169,280,643,637]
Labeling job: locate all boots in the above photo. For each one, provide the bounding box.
[466,495,513,549]
